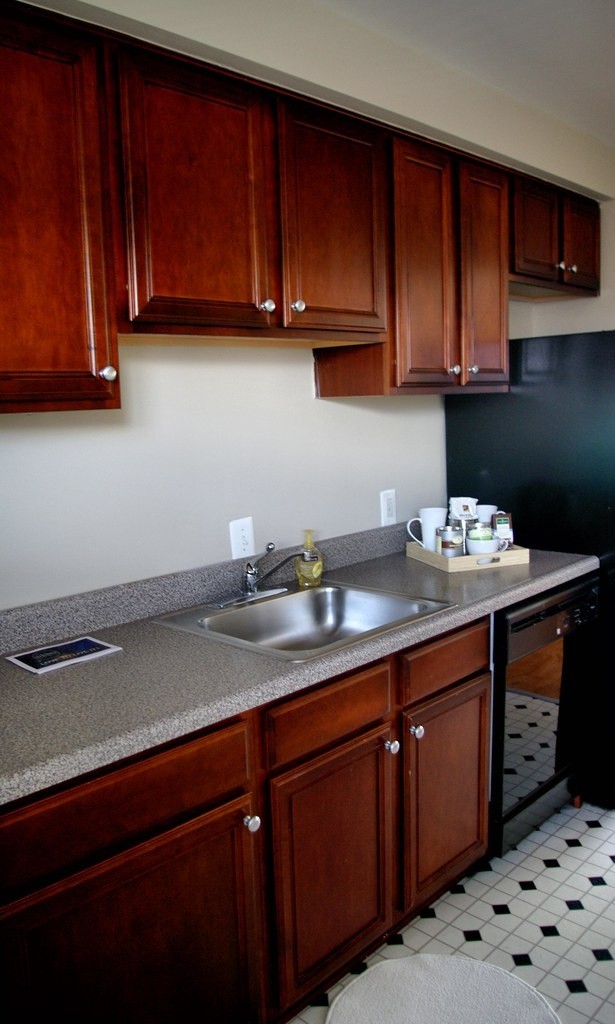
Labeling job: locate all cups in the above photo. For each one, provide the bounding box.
[448,518,478,554]
[466,536,508,555]
[436,526,464,558]
[476,505,506,522]
[406,508,448,550]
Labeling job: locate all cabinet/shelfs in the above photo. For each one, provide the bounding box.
[250,616,491,1015]
[0,718,263,1024]
[311,137,511,396]
[509,174,601,305]
[112,41,386,349]
[0,24,123,413]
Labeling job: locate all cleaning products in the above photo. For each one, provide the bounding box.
[296,529,324,587]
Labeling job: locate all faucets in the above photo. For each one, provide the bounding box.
[245,543,309,596]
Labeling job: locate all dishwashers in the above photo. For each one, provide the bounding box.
[494,578,601,858]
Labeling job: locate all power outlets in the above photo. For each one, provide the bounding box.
[229,515,256,561]
[380,489,397,526]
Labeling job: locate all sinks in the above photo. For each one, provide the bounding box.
[199,585,458,664]
